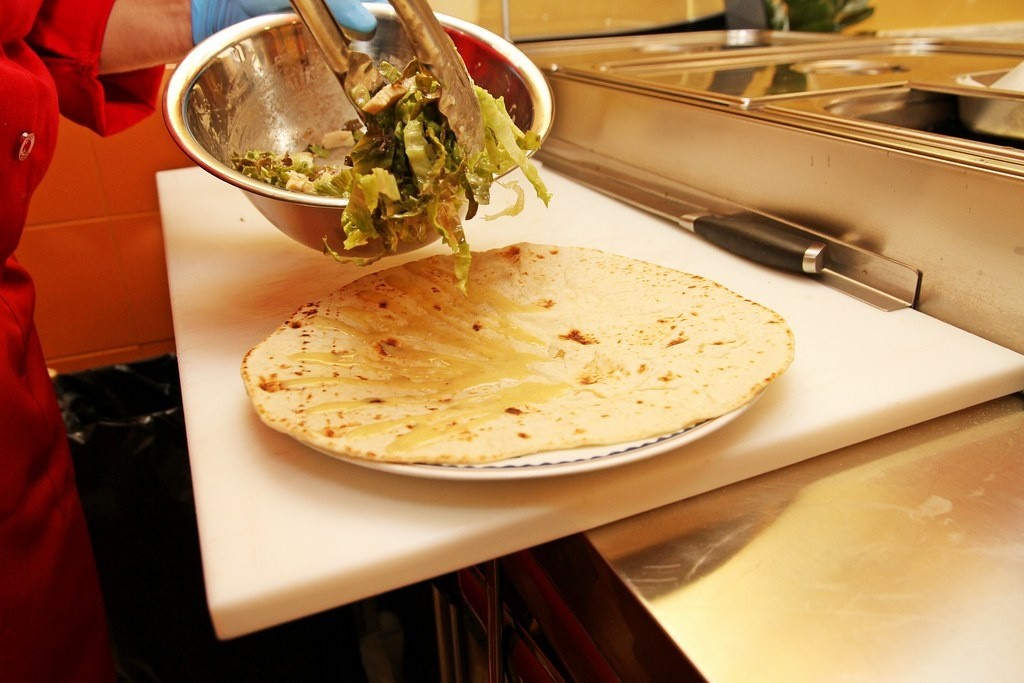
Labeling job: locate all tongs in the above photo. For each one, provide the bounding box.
[290,0,484,146]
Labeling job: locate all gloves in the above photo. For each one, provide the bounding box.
[191,0,388,46]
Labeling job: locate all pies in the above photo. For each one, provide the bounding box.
[242,240,797,463]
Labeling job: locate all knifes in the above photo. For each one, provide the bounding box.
[533,152,825,276]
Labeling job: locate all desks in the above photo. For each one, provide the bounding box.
[156,161,1024,642]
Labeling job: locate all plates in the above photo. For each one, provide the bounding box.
[289,385,772,482]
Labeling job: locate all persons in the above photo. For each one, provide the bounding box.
[1,0,377,683]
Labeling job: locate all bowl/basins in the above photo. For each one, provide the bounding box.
[160,0,556,260]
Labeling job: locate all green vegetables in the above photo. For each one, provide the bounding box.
[231,51,553,296]
